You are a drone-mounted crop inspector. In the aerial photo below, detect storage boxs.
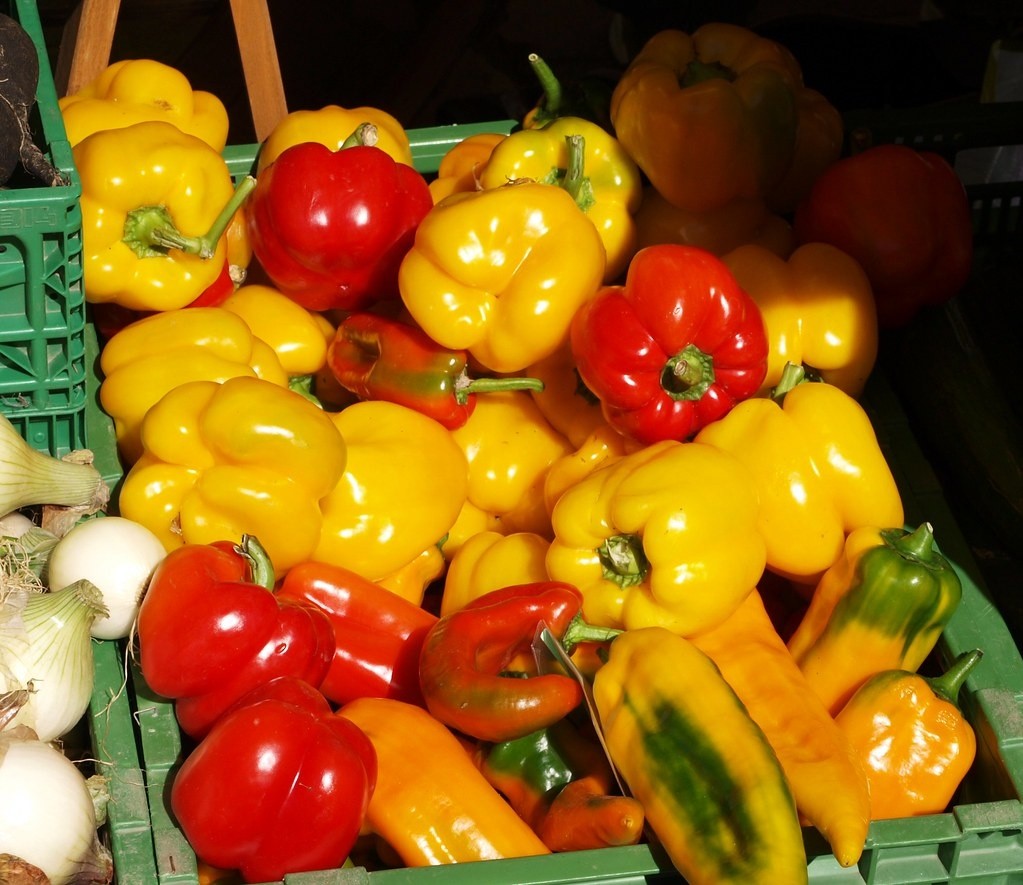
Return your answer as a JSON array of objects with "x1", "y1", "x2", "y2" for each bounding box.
[{"x1": 1, "y1": 1, "x2": 1023, "y2": 885}]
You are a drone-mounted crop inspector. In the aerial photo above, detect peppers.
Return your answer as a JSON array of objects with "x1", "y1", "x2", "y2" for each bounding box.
[{"x1": 50, "y1": 19, "x2": 982, "y2": 885}]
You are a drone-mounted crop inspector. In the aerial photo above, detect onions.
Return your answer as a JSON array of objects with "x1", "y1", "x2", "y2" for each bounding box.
[{"x1": 0, "y1": 413, "x2": 173, "y2": 885}]
